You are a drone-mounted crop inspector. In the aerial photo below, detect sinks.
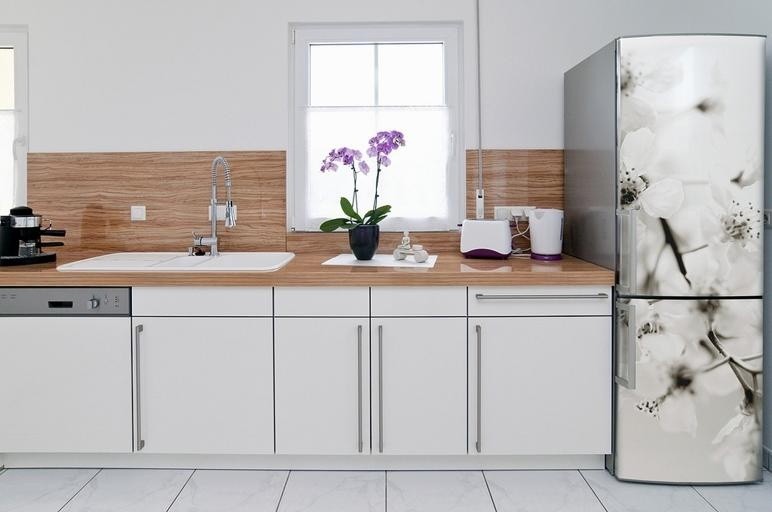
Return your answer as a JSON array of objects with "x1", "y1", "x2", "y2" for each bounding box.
[{"x1": 55, "y1": 251, "x2": 296, "y2": 274}]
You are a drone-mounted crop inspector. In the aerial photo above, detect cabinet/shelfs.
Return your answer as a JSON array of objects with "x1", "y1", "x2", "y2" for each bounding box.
[
  {"x1": 0, "y1": 270, "x2": 127, "y2": 470},
  {"x1": 273, "y1": 272, "x2": 466, "y2": 459},
  {"x1": 128, "y1": 271, "x2": 278, "y2": 463},
  {"x1": 468, "y1": 273, "x2": 614, "y2": 459}
]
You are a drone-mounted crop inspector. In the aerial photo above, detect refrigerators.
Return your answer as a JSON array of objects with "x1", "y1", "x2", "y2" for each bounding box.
[{"x1": 563, "y1": 34, "x2": 766, "y2": 487}]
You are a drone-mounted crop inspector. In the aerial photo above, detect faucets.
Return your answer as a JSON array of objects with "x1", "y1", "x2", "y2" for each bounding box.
[{"x1": 189, "y1": 155, "x2": 235, "y2": 257}]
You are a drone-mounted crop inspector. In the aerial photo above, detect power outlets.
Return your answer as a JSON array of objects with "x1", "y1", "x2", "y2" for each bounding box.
[
  {"x1": 509, "y1": 207, "x2": 523, "y2": 222},
  {"x1": 130, "y1": 206, "x2": 147, "y2": 221},
  {"x1": 494, "y1": 207, "x2": 510, "y2": 221},
  {"x1": 209, "y1": 205, "x2": 238, "y2": 221},
  {"x1": 522, "y1": 206, "x2": 536, "y2": 221}
]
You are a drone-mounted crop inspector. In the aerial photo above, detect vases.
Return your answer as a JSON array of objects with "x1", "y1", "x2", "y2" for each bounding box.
[{"x1": 348, "y1": 225, "x2": 380, "y2": 261}]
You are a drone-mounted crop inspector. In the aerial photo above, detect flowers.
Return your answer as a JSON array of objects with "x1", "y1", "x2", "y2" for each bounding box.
[{"x1": 318, "y1": 131, "x2": 406, "y2": 232}]
